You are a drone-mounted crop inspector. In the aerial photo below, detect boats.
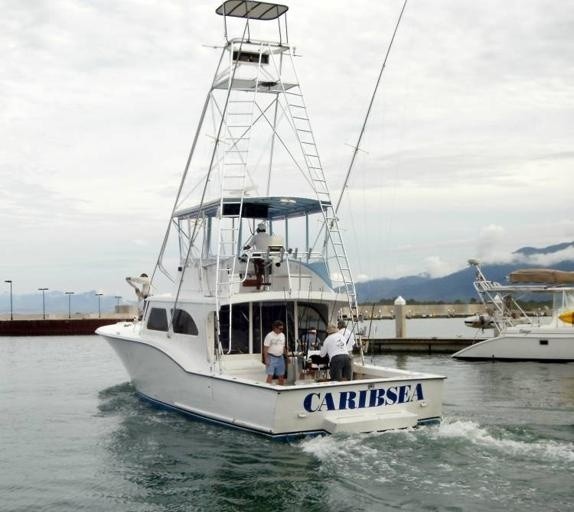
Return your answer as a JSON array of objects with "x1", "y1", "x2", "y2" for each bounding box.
[
  {"x1": 93, "y1": 0, "x2": 449, "y2": 444},
  {"x1": 450, "y1": 258, "x2": 574, "y2": 363}
]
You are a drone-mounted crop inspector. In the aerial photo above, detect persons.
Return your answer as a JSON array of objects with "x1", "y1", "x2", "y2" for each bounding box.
[
  {"x1": 337, "y1": 319, "x2": 357, "y2": 380},
  {"x1": 320, "y1": 323, "x2": 353, "y2": 381},
  {"x1": 244, "y1": 222, "x2": 272, "y2": 288},
  {"x1": 301, "y1": 327, "x2": 321, "y2": 353},
  {"x1": 125, "y1": 273, "x2": 151, "y2": 312},
  {"x1": 264, "y1": 320, "x2": 288, "y2": 385}
]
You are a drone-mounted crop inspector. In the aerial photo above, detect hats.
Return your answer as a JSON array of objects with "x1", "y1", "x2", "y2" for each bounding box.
[
  {"x1": 257, "y1": 223, "x2": 267, "y2": 230},
  {"x1": 337, "y1": 320, "x2": 347, "y2": 328},
  {"x1": 326, "y1": 324, "x2": 339, "y2": 334}
]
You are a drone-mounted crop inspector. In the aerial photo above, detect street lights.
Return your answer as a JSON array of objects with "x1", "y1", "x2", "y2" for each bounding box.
[{"x1": 4, "y1": 280, "x2": 123, "y2": 320}]
